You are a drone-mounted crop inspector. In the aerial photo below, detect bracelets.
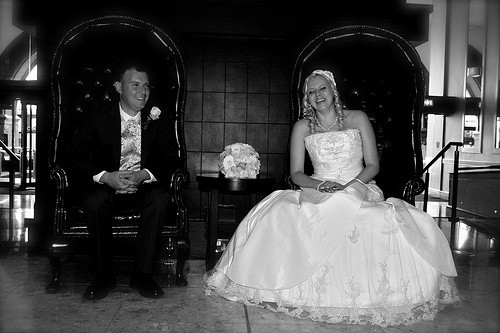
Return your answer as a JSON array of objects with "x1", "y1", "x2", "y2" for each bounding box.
[{"x1": 316, "y1": 181, "x2": 326, "y2": 191}]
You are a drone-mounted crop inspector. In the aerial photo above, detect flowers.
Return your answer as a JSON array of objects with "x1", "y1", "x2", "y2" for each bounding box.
[{"x1": 218, "y1": 142, "x2": 261, "y2": 179}]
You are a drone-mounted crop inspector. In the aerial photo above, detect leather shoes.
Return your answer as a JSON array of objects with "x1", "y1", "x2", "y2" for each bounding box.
[
  {"x1": 128, "y1": 278, "x2": 164, "y2": 299},
  {"x1": 83, "y1": 277, "x2": 116, "y2": 301}
]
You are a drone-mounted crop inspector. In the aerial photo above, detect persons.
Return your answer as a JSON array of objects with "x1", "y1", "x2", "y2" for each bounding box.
[
  {"x1": 206, "y1": 70, "x2": 460, "y2": 327},
  {"x1": 79, "y1": 66, "x2": 173, "y2": 303}
]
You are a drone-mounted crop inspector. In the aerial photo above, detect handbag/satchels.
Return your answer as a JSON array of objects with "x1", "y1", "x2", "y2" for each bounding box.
[{"x1": 367, "y1": 180, "x2": 385, "y2": 202}]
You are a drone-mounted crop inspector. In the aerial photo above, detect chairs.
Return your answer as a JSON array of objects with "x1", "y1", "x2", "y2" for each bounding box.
[
  {"x1": 278, "y1": 22, "x2": 426, "y2": 206},
  {"x1": 33, "y1": 15, "x2": 191, "y2": 287}
]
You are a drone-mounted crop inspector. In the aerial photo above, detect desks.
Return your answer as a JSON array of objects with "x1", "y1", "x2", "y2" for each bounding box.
[{"x1": 196, "y1": 172, "x2": 275, "y2": 278}]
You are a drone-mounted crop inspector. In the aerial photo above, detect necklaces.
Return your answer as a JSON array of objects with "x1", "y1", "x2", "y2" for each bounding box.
[{"x1": 316, "y1": 117, "x2": 338, "y2": 131}]
